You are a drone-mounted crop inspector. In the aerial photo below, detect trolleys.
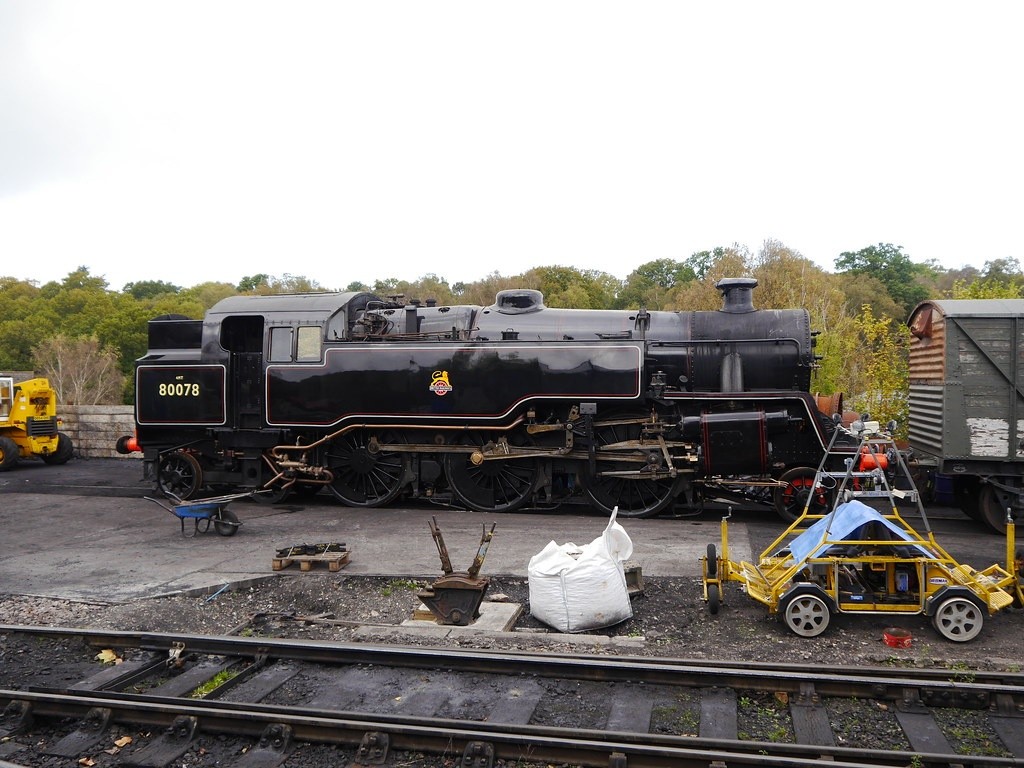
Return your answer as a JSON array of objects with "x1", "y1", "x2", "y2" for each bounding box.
[{"x1": 143, "y1": 487, "x2": 273, "y2": 535}]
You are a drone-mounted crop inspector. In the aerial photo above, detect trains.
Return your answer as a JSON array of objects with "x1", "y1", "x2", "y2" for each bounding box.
[{"x1": 115, "y1": 277, "x2": 1024, "y2": 526}]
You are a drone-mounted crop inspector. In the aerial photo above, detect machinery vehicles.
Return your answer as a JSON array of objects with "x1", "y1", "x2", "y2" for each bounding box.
[{"x1": 0, "y1": 370, "x2": 76, "y2": 473}]
